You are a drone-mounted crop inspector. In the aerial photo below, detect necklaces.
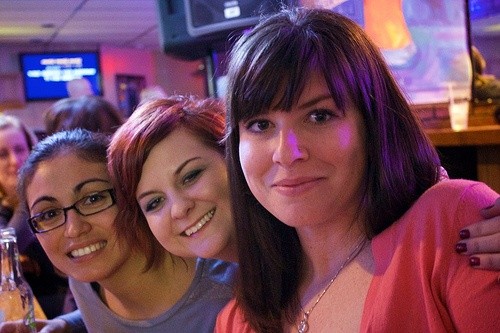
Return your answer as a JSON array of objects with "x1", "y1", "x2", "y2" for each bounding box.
[{"x1": 293, "y1": 237, "x2": 366, "y2": 333}]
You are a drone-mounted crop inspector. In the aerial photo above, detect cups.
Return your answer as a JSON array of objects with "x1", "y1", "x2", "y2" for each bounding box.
[{"x1": 449, "y1": 82, "x2": 470, "y2": 131}]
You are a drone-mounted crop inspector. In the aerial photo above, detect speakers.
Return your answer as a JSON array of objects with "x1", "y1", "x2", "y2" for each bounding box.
[{"x1": 157, "y1": 0, "x2": 300, "y2": 62}]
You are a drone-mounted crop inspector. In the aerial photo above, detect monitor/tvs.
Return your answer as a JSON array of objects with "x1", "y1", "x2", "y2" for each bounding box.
[{"x1": 19, "y1": 50, "x2": 103, "y2": 102}]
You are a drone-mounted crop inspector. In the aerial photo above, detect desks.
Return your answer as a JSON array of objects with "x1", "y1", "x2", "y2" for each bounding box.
[
  {"x1": 425, "y1": 124, "x2": 500, "y2": 194},
  {"x1": 0, "y1": 255, "x2": 48, "y2": 321}
]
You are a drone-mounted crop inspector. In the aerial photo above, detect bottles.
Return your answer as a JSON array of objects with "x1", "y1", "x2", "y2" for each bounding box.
[{"x1": 0, "y1": 228, "x2": 36, "y2": 333}]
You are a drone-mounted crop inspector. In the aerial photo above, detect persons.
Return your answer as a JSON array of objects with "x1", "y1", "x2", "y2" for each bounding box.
[
  {"x1": 0, "y1": 95, "x2": 500, "y2": 333},
  {"x1": 213, "y1": 5, "x2": 500, "y2": 333}
]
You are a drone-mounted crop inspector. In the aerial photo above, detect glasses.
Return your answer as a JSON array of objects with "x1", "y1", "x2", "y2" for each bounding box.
[{"x1": 25, "y1": 186, "x2": 121, "y2": 234}]
